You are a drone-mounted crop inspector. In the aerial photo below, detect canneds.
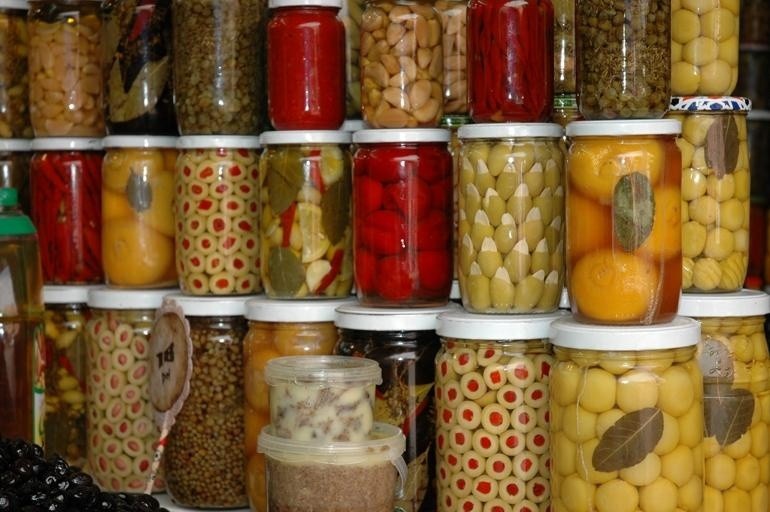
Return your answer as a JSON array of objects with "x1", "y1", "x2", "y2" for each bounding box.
[{"x1": 0, "y1": 0, "x2": 770, "y2": 511}]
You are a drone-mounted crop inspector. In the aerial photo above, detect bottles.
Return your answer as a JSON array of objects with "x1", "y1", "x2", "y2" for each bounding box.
[{"x1": 1, "y1": 184, "x2": 49, "y2": 457}]
